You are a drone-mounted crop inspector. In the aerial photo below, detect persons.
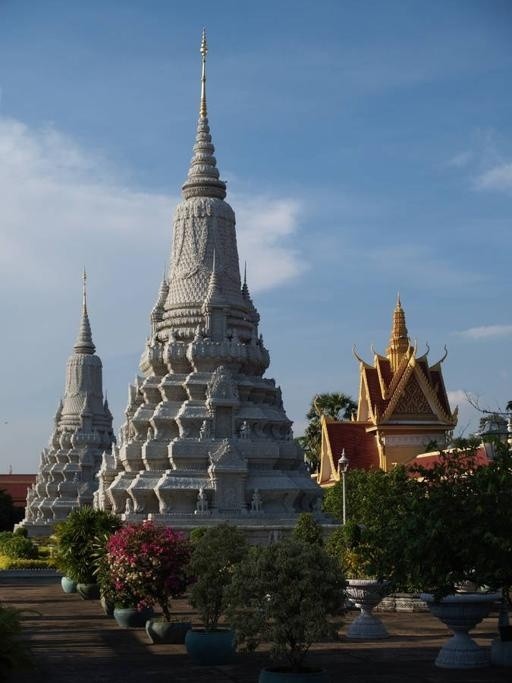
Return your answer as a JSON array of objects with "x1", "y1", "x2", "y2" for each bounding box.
[
  {"x1": 240, "y1": 421, "x2": 249, "y2": 438},
  {"x1": 251, "y1": 489, "x2": 263, "y2": 511},
  {"x1": 200, "y1": 420, "x2": 210, "y2": 438},
  {"x1": 197, "y1": 488, "x2": 209, "y2": 512}
]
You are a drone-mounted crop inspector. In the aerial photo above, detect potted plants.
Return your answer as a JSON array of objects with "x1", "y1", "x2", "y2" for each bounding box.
[
  {"x1": 47, "y1": 511, "x2": 345, "y2": 681},
  {"x1": 325, "y1": 438, "x2": 511, "y2": 669}
]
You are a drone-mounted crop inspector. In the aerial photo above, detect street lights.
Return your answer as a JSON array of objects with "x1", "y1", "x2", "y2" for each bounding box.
[
  {"x1": 478, "y1": 411, "x2": 512, "y2": 471},
  {"x1": 335, "y1": 447, "x2": 352, "y2": 525}
]
